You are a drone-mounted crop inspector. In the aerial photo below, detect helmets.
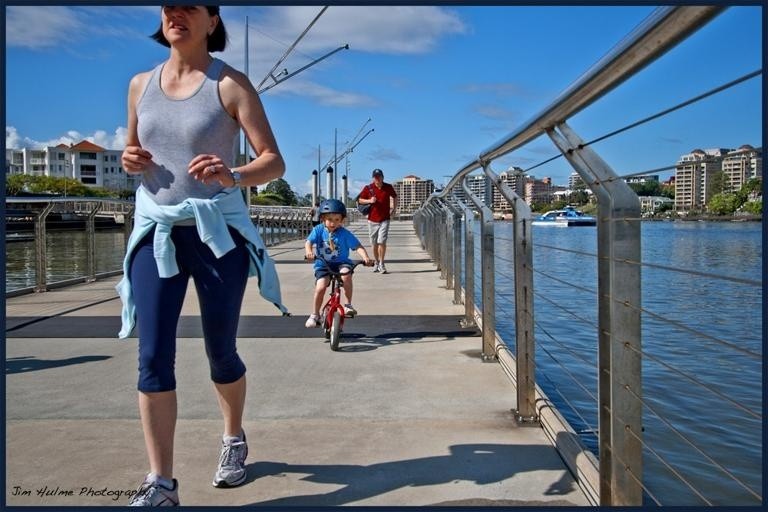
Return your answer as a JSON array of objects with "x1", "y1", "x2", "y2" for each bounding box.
[{"x1": 319, "y1": 199, "x2": 347, "y2": 220}]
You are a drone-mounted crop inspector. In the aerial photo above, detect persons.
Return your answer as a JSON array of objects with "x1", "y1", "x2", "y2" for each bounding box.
[
  {"x1": 310, "y1": 202, "x2": 321, "y2": 228},
  {"x1": 121, "y1": 2, "x2": 286, "y2": 507},
  {"x1": 355, "y1": 169, "x2": 397, "y2": 274},
  {"x1": 304, "y1": 199, "x2": 371, "y2": 328}
]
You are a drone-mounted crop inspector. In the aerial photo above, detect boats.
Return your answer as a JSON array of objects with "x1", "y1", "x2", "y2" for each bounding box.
[{"x1": 528, "y1": 206, "x2": 597, "y2": 228}]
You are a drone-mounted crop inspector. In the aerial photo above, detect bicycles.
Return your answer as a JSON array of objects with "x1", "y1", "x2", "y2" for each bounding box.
[{"x1": 304, "y1": 255, "x2": 375, "y2": 352}]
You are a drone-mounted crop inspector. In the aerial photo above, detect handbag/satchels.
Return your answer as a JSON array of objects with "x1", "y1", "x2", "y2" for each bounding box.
[{"x1": 355, "y1": 197, "x2": 373, "y2": 214}]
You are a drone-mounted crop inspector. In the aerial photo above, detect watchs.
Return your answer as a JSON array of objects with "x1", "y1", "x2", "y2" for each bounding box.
[{"x1": 228, "y1": 167, "x2": 241, "y2": 188}]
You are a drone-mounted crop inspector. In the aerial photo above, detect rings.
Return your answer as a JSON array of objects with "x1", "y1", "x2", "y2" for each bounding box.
[{"x1": 210, "y1": 165, "x2": 216, "y2": 174}]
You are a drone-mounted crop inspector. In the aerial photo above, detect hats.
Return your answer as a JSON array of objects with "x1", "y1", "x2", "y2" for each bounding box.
[{"x1": 372, "y1": 170, "x2": 383, "y2": 177}]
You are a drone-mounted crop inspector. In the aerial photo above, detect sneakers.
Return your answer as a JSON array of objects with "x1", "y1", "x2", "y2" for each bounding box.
[
  {"x1": 214, "y1": 429, "x2": 248, "y2": 487},
  {"x1": 372, "y1": 262, "x2": 387, "y2": 274},
  {"x1": 305, "y1": 314, "x2": 320, "y2": 328},
  {"x1": 344, "y1": 304, "x2": 357, "y2": 315},
  {"x1": 130, "y1": 479, "x2": 180, "y2": 507}
]
